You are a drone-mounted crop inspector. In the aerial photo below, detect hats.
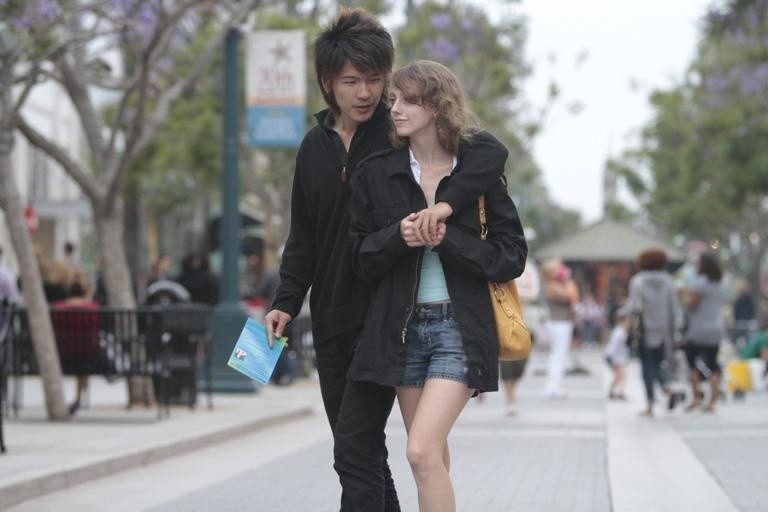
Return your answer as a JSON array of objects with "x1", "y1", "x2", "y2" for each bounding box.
[{"x1": 539, "y1": 259, "x2": 571, "y2": 283}]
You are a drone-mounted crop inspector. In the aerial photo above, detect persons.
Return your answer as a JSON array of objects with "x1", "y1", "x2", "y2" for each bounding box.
[
  {"x1": 262, "y1": 5, "x2": 511, "y2": 511},
  {"x1": 151, "y1": 249, "x2": 279, "y2": 304},
  {"x1": 338, "y1": 59, "x2": 532, "y2": 510},
  {"x1": 0, "y1": 243, "x2": 115, "y2": 413},
  {"x1": 500, "y1": 250, "x2": 768, "y2": 412}
]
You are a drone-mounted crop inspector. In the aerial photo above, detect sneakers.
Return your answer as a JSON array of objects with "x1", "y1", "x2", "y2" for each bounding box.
[{"x1": 605, "y1": 388, "x2": 719, "y2": 418}]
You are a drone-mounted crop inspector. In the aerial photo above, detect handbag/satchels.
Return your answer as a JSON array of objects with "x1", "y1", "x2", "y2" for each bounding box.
[
  {"x1": 475, "y1": 191, "x2": 534, "y2": 364},
  {"x1": 628, "y1": 313, "x2": 646, "y2": 357}
]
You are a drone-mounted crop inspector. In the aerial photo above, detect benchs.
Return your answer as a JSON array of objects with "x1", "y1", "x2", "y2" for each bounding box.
[
  {"x1": 270, "y1": 313, "x2": 313, "y2": 384},
  {"x1": 2, "y1": 306, "x2": 219, "y2": 418}
]
